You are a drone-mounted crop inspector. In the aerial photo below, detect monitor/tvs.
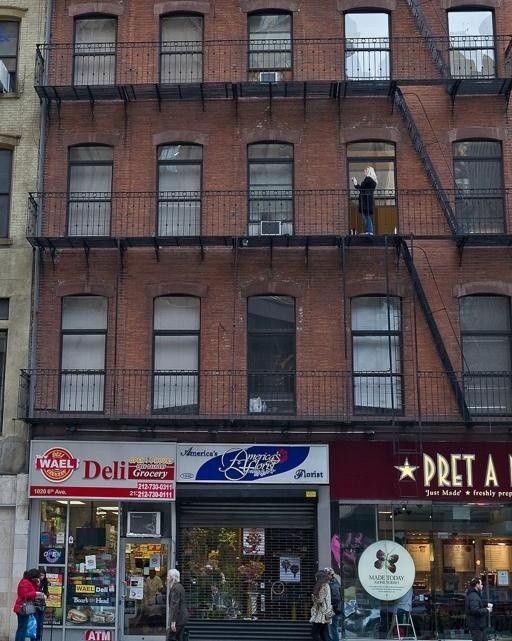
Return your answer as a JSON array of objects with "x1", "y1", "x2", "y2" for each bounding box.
[
  {"x1": 125, "y1": 512, "x2": 162, "y2": 537},
  {"x1": 76, "y1": 526, "x2": 106, "y2": 547}
]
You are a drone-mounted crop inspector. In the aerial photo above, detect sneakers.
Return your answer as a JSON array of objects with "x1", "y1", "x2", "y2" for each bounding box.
[{"x1": 359, "y1": 231, "x2": 374, "y2": 236}]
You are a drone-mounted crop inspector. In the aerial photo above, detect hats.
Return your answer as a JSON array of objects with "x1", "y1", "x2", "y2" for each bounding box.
[{"x1": 324, "y1": 567, "x2": 334, "y2": 575}]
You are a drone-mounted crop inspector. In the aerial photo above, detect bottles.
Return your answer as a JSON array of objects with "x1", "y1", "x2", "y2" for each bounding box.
[{"x1": 393, "y1": 226, "x2": 398, "y2": 234}]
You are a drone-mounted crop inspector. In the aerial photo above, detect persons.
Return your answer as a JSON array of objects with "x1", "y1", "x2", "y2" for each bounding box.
[
  {"x1": 309, "y1": 571, "x2": 336, "y2": 641},
  {"x1": 146, "y1": 570, "x2": 165, "y2": 633},
  {"x1": 351, "y1": 166, "x2": 378, "y2": 235},
  {"x1": 30, "y1": 567, "x2": 50, "y2": 641},
  {"x1": 397, "y1": 586, "x2": 413, "y2": 624},
  {"x1": 166, "y1": 569, "x2": 189, "y2": 641},
  {"x1": 13, "y1": 568, "x2": 47, "y2": 641},
  {"x1": 465, "y1": 577, "x2": 493, "y2": 641},
  {"x1": 324, "y1": 567, "x2": 345, "y2": 641}
]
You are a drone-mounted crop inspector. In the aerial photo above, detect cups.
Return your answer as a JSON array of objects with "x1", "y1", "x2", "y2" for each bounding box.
[{"x1": 488, "y1": 602, "x2": 494, "y2": 608}]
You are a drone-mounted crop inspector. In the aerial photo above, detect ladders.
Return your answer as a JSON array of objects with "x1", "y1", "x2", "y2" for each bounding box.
[{"x1": 395, "y1": 613, "x2": 417, "y2": 641}]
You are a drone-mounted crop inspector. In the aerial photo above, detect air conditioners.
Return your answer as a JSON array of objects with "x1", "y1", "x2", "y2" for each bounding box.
[
  {"x1": 260, "y1": 72, "x2": 278, "y2": 85},
  {"x1": 260, "y1": 221, "x2": 281, "y2": 235}
]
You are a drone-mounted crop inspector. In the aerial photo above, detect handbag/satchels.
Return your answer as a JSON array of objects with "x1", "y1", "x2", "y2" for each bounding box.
[
  {"x1": 25, "y1": 614, "x2": 37, "y2": 639},
  {"x1": 21, "y1": 602, "x2": 36, "y2": 615}
]
